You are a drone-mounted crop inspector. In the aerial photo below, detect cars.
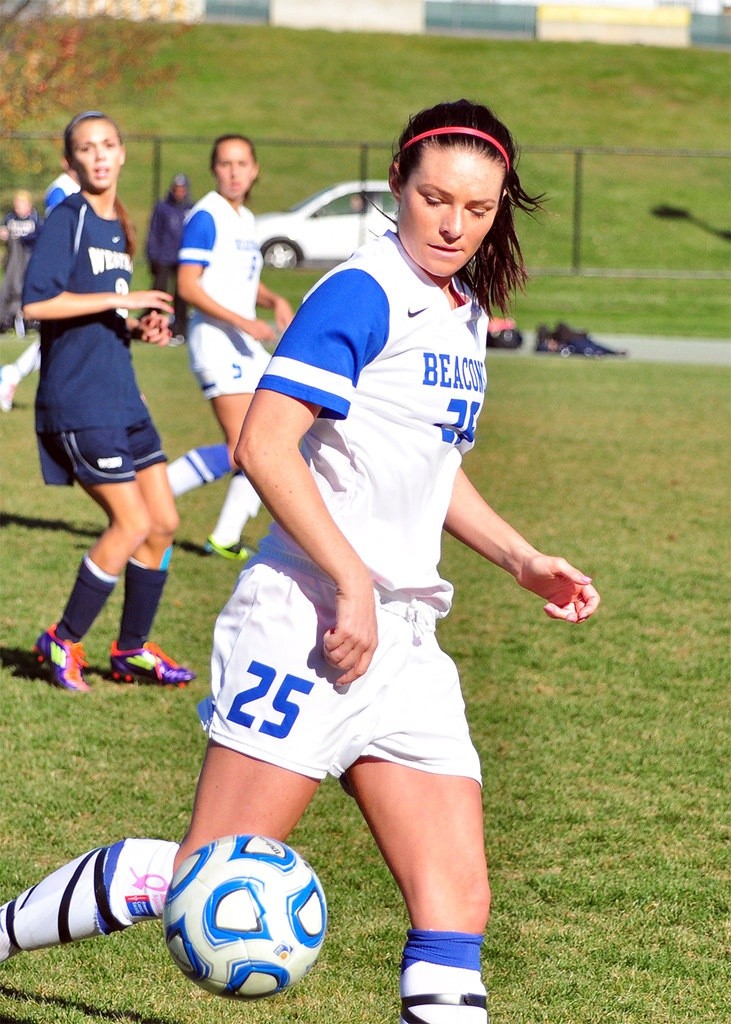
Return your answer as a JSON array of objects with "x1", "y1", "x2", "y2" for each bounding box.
[{"x1": 253, "y1": 179, "x2": 399, "y2": 268}]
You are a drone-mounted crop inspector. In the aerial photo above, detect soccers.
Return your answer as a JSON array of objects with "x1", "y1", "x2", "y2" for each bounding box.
[{"x1": 164, "y1": 834, "x2": 325, "y2": 999}]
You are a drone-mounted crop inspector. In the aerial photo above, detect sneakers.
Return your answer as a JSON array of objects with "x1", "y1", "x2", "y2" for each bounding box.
[
  {"x1": 205, "y1": 536, "x2": 255, "y2": 562},
  {"x1": 110, "y1": 640, "x2": 196, "y2": 686},
  {"x1": 34, "y1": 624, "x2": 88, "y2": 690},
  {"x1": 1, "y1": 364, "x2": 21, "y2": 413}
]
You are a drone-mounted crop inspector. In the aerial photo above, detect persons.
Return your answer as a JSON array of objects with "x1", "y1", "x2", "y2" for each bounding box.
[
  {"x1": 0, "y1": 148, "x2": 82, "y2": 413},
  {"x1": 0, "y1": 188, "x2": 41, "y2": 331},
  {"x1": 146, "y1": 172, "x2": 196, "y2": 341},
  {"x1": 349, "y1": 193, "x2": 363, "y2": 213},
  {"x1": 164, "y1": 136, "x2": 295, "y2": 563},
  {"x1": 0, "y1": 98, "x2": 602, "y2": 1024},
  {"x1": 21, "y1": 110, "x2": 196, "y2": 692}
]
